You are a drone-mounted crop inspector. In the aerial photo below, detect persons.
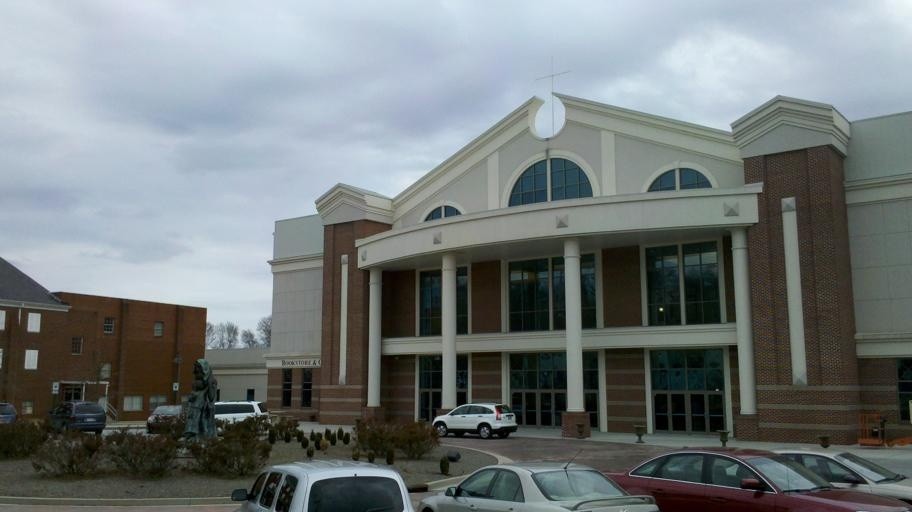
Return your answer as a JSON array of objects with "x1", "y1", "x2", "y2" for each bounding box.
[{"x1": 181, "y1": 359, "x2": 218, "y2": 442}]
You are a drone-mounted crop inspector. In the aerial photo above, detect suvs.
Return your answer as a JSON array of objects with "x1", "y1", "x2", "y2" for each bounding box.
[
  {"x1": 426, "y1": 401, "x2": 519, "y2": 439},
  {"x1": 46, "y1": 400, "x2": 106, "y2": 437}
]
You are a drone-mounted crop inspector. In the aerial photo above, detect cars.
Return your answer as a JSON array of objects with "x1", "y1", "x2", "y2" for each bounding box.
[
  {"x1": 776, "y1": 446, "x2": 912, "y2": 505},
  {"x1": 602, "y1": 445, "x2": 912, "y2": 510},
  {"x1": 0, "y1": 402, "x2": 20, "y2": 424},
  {"x1": 416, "y1": 449, "x2": 658, "y2": 511},
  {"x1": 148, "y1": 405, "x2": 184, "y2": 434}
]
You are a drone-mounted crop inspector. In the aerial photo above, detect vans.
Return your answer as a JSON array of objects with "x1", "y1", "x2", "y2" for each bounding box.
[
  {"x1": 214, "y1": 399, "x2": 271, "y2": 436},
  {"x1": 228, "y1": 458, "x2": 430, "y2": 512}
]
